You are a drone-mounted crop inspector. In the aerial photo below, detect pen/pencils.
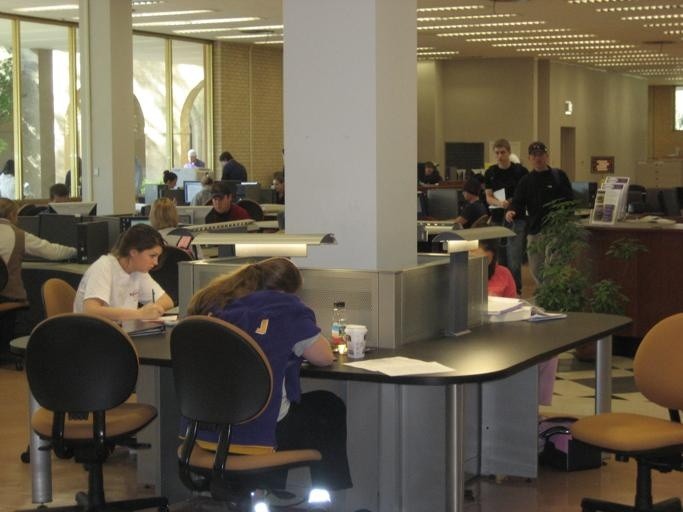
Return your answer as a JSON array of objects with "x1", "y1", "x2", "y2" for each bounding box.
[{"x1": 152, "y1": 289, "x2": 155, "y2": 303}]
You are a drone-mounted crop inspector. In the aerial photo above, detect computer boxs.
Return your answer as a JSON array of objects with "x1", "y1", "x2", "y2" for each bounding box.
[
  {"x1": 427, "y1": 189, "x2": 463, "y2": 218},
  {"x1": 77, "y1": 221, "x2": 107, "y2": 264},
  {"x1": 145, "y1": 184, "x2": 169, "y2": 204}
]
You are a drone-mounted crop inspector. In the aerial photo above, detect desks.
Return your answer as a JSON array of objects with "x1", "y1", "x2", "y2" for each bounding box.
[{"x1": 10, "y1": 312, "x2": 639, "y2": 512}]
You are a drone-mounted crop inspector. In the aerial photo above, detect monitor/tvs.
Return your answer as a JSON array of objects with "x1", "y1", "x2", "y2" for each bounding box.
[
  {"x1": 38, "y1": 214, "x2": 79, "y2": 246},
  {"x1": 184, "y1": 180, "x2": 203, "y2": 203},
  {"x1": 241, "y1": 181, "x2": 261, "y2": 189},
  {"x1": 49, "y1": 202, "x2": 98, "y2": 218},
  {"x1": 160, "y1": 189, "x2": 187, "y2": 206},
  {"x1": 445, "y1": 141, "x2": 484, "y2": 170},
  {"x1": 417, "y1": 192, "x2": 424, "y2": 215},
  {"x1": 120, "y1": 217, "x2": 151, "y2": 234}
]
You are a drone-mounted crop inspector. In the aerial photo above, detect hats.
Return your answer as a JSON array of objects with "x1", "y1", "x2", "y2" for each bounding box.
[
  {"x1": 208, "y1": 184, "x2": 228, "y2": 199},
  {"x1": 528, "y1": 142, "x2": 548, "y2": 153}
]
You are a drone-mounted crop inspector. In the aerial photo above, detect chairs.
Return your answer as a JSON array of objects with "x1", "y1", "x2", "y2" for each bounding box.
[
  {"x1": 20, "y1": 278, "x2": 137, "y2": 464},
  {"x1": 570, "y1": 311, "x2": 683, "y2": 511},
  {"x1": 23, "y1": 312, "x2": 169, "y2": 512},
  {"x1": 0, "y1": 182, "x2": 285, "y2": 371},
  {"x1": 417, "y1": 169, "x2": 683, "y2": 235},
  {"x1": 170, "y1": 316, "x2": 322, "y2": 511}
]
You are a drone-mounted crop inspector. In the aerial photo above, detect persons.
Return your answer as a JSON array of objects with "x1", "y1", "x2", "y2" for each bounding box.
[
  {"x1": 177, "y1": 256, "x2": 354, "y2": 512},
  {"x1": 417, "y1": 161, "x2": 442, "y2": 187},
  {"x1": 190, "y1": 176, "x2": 214, "y2": 206},
  {"x1": 49, "y1": 183, "x2": 70, "y2": 203},
  {"x1": 0, "y1": 159, "x2": 16, "y2": 199},
  {"x1": 147, "y1": 197, "x2": 179, "y2": 245},
  {"x1": 271, "y1": 170, "x2": 284, "y2": 204},
  {"x1": 72, "y1": 223, "x2": 173, "y2": 327},
  {"x1": 219, "y1": 151, "x2": 247, "y2": 183},
  {"x1": 162, "y1": 170, "x2": 177, "y2": 189},
  {"x1": 0, "y1": 199, "x2": 82, "y2": 347},
  {"x1": 64, "y1": 156, "x2": 81, "y2": 195},
  {"x1": 454, "y1": 138, "x2": 572, "y2": 298},
  {"x1": 204, "y1": 180, "x2": 250, "y2": 224},
  {"x1": 183, "y1": 149, "x2": 205, "y2": 168}
]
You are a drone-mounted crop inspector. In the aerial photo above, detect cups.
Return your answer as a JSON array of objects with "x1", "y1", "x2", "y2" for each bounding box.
[
  {"x1": 345, "y1": 322, "x2": 369, "y2": 359},
  {"x1": 457, "y1": 168, "x2": 468, "y2": 181}
]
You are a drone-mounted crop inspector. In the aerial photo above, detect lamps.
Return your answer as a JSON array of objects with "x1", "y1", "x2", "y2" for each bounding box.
[
  {"x1": 191, "y1": 232, "x2": 336, "y2": 259},
  {"x1": 430, "y1": 226, "x2": 517, "y2": 336}
]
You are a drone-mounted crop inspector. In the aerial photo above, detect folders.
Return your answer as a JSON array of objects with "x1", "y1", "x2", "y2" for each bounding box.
[{"x1": 120, "y1": 320, "x2": 166, "y2": 337}]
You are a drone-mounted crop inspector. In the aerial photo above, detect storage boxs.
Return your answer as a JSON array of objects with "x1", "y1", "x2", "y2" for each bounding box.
[{"x1": 538, "y1": 417, "x2": 601, "y2": 472}]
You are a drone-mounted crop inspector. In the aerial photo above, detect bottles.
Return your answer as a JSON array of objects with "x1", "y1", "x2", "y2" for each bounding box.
[{"x1": 331, "y1": 299, "x2": 346, "y2": 351}]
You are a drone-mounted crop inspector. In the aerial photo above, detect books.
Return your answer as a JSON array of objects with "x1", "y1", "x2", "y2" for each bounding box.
[
  {"x1": 600, "y1": 181, "x2": 625, "y2": 220},
  {"x1": 606, "y1": 176, "x2": 630, "y2": 218},
  {"x1": 591, "y1": 189, "x2": 620, "y2": 225},
  {"x1": 485, "y1": 295, "x2": 531, "y2": 315},
  {"x1": 488, "y1": 305, "x2": 547, "y2": 321},
  {"x1": 121, "y1": 317, "x2": 166, "y2": 336},
  {"x1": 529, "y1": 312, "x2": 567, "y2": 321}
]
